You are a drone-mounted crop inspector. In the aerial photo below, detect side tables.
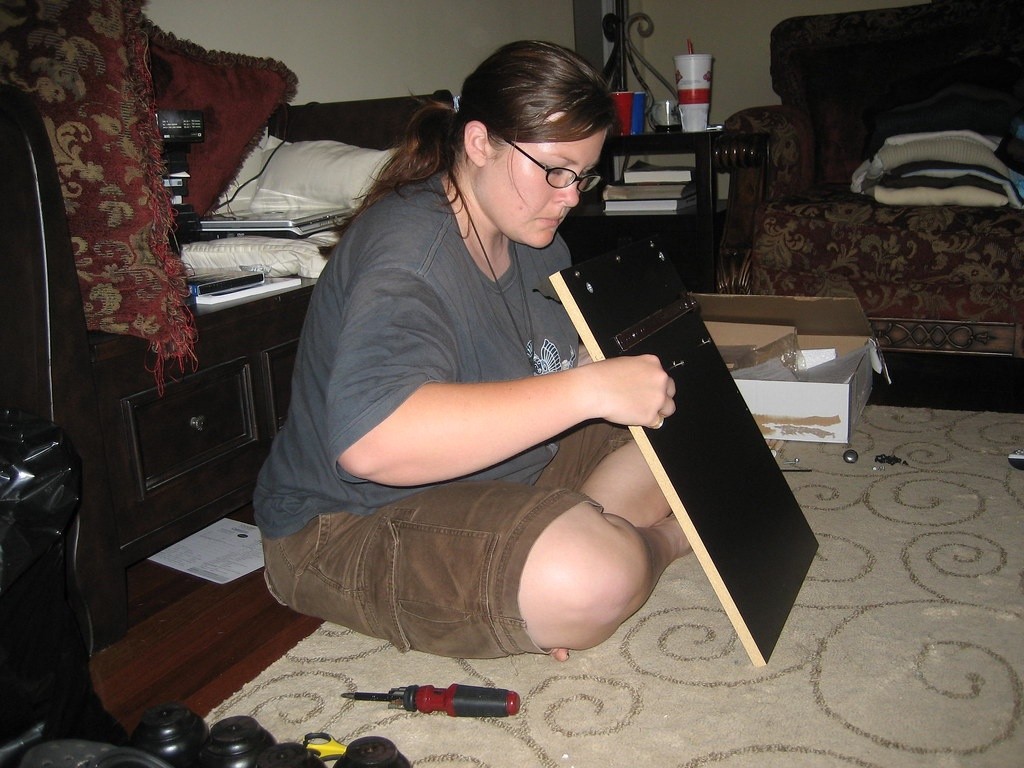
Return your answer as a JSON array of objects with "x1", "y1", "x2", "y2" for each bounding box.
[{"x1": 558, "y1": 131, "x2": 725, "y2": 293}]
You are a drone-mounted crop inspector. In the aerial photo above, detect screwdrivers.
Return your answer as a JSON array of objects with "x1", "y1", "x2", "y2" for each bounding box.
[{"x1": 340, "y1": 683, "x2": 522, "y2": 719}]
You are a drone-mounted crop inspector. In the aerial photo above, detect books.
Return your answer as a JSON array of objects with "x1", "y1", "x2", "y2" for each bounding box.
[{"x1": 603, "y1": 161, "x2": 697, "y2": 211}]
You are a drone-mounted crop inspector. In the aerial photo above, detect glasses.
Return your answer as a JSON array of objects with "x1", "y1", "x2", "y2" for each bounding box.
[{"x1": 489, "y1": 125, "x2": 602, "y2": 192}]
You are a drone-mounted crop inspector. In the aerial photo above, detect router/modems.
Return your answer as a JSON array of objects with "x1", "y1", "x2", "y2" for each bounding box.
[{"x1": 187, "y1": 269, "x2": 264, "y2": 297}]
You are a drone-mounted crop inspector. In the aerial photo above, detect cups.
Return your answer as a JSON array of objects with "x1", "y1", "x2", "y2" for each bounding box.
[
  {"x1": 649, "y1": 101, "x2": 681, "y2": 131},
  {"x1": 673, "y1": 53, "x2": 712, "y2": 133},
  {"x1": 608, "y1": 91, "x2": 634, "y2": 136},
  {"x1": 629, "y1": 91, "x2": 654, "y2": 135}
]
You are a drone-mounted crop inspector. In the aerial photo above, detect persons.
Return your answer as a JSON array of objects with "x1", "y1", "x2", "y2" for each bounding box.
[{"x1": 252, "y1": 39, "x2": 691, "y2": 658}]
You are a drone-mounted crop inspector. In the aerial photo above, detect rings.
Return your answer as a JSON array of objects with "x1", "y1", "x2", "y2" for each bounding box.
[{"x1": 651, "y1": 417, "x2": 663, "y2": 428}]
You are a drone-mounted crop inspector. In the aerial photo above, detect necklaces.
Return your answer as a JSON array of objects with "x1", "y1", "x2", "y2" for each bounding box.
[{"x1": 456, "y1": 184, "x2": 536, "y2": 373}]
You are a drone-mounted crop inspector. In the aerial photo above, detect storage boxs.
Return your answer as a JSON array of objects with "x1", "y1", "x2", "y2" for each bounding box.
[{"x1": 704, "y1": 321, "x2": 874, "y2": 445}]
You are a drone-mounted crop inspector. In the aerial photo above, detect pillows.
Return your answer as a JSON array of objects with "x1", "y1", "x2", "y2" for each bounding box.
[
  {"x1": 144, "y1": 21, "x2": 300, "y2": 220},
  {"x1": 250, "y1": 138, "x2": 397, "y2": 209},
  {"x1": 0, "y1": 0, "x2": 200, "y2": 397}
]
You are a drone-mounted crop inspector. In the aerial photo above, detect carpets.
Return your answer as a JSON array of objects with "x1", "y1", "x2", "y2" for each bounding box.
[{"x1": 204, "y1": 404, "x2": 1024, "y2": 768}]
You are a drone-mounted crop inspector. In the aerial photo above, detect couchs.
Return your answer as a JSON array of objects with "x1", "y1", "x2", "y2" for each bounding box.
[
  {"x1": 0, "y1": 85, "x2": 454, "y2": 661},
  {"x1": 712, "y1": 0, "x2": 1024, "y2": 413}
]
